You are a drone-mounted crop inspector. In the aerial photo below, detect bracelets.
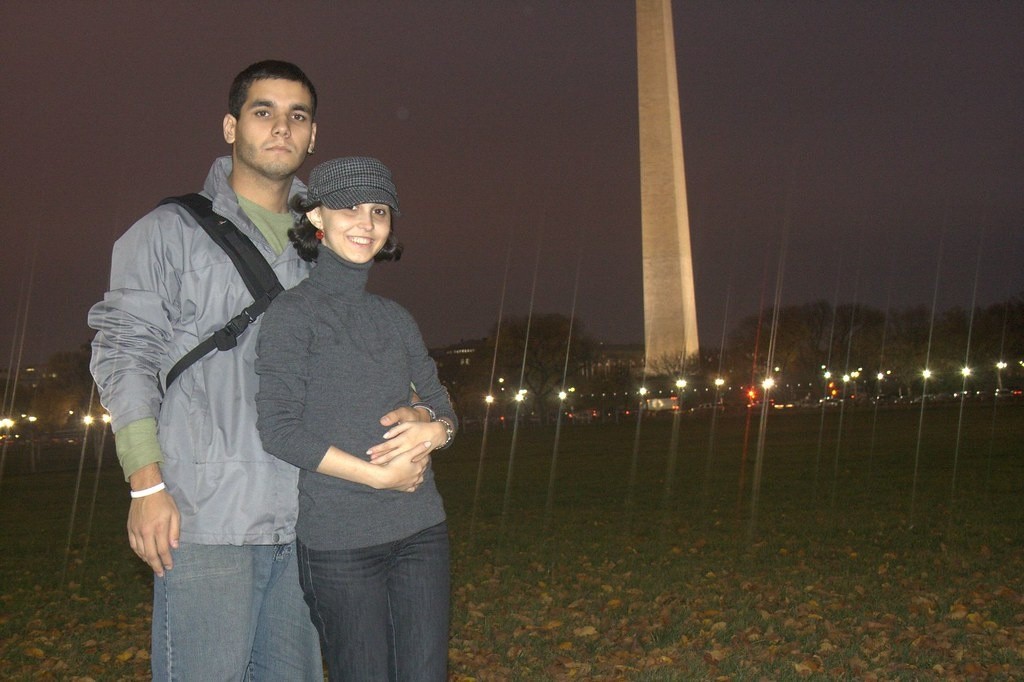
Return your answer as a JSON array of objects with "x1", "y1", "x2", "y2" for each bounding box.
[
  {"x1": 412, "y1": 403, "x2": 436, "y2": 419},
  {"x1": 435, "y1": 419, "x2": 453, "y2": 450},
  {"x1": 131, "y1": 483, "x2": 166, "y2": 498}
]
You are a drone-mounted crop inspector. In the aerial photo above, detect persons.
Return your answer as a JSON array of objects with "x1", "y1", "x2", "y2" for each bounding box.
[
  {"x1": 86, "y1": 61, "x2": 432, "y2": 681},
  {"x1": 255, "y1": 157, "x2": 460, "y2": 682}
]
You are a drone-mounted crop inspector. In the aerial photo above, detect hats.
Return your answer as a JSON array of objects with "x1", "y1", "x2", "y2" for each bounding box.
[{"x1": 305, "y1": 156, "x2": 401, "y2": 217}]
